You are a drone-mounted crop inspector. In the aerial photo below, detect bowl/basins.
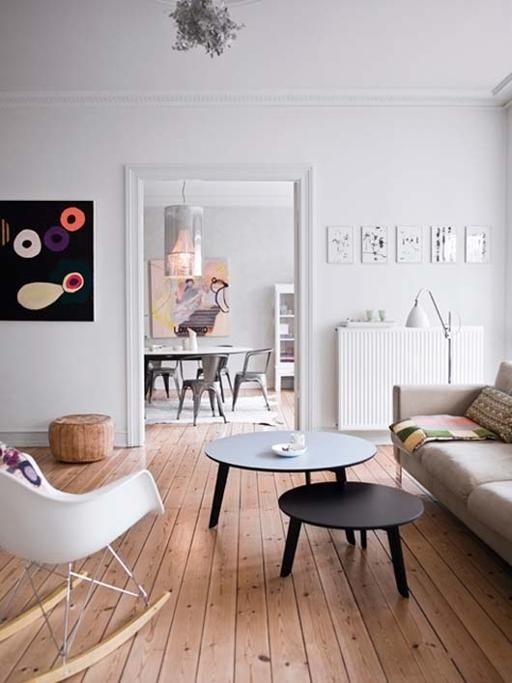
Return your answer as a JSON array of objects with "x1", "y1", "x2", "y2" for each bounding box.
[{"x1": 173, "y1": 345, "x2": 182, "y2": 350}]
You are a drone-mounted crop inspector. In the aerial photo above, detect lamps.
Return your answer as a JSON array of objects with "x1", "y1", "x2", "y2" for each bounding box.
[
  {"x1": 164, "y1": 180, "x2": 203, "y2": 279},
  {"x1": 405, "y1": 287, "x2": 452, "y2": 384}
]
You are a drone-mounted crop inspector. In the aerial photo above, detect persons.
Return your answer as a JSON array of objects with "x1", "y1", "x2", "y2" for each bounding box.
[{"x1": 172, "y1": 279, "x2": 202, "y2": 326}]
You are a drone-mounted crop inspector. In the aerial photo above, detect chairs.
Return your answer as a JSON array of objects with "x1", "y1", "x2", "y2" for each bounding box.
[
  {"x1": 144, "y1": 345, "x2": 273, "y2": 426},
  {"x1": 0, "y1": 452, "x2": 171, "y2": 683}
]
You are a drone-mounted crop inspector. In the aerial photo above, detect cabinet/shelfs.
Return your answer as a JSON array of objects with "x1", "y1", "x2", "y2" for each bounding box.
[{"x1": 274, "y1": 283, "x2": 295, "y2": 393}]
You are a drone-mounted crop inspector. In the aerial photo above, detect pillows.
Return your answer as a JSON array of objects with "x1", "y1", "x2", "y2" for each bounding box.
[{"x1": 463, "y1": 387, "x2": 512, "y2": 443}]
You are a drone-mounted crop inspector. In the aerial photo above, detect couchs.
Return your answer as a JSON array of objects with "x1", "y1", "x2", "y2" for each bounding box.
[{"x1": 392, "y1": 361, "x2": 512, "y2": 568}]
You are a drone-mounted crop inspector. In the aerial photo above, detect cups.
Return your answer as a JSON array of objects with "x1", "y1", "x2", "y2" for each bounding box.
[
  {"x1": 289, "y1": 327, "x2": 293, "y2": 337},
  {"x1": 287, "y1": 432, "x2": 305, "y2": 449},
  {"x1": 288, "y1": 304, "x2": 293, "y2": 314},
  {"x1": 280, "y1": 304, "x2": 288, "y2": 315},
  {"x1": 183, "y1": 338, "x2": 189, "y2": 350},
  {"x1": 366, "y1": 310, "x2": 386, "y2": 322}
]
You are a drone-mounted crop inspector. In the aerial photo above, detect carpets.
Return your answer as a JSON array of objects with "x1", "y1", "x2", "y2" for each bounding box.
[{"x1": 144, "y1": 393, "x2": 287, "y2": 429}]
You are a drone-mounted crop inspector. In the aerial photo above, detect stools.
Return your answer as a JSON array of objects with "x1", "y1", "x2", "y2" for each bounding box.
[{"x1": 49, "y1": 413, "x2": 116, "y2": 461}]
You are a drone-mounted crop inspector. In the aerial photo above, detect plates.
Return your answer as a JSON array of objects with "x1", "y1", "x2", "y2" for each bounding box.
[{"x1": 272, "y1": 444, "x2": 308, "y2": 457}]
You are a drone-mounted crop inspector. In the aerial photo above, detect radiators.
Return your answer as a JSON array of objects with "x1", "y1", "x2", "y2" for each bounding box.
[{"x1": 337, "y1": 326, "x2": 450, "y2": 427}]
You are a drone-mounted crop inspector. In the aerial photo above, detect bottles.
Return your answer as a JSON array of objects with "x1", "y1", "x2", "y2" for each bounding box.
[{"x1": 189, "y1": 331, "x2": 198, "y2": 351}]
